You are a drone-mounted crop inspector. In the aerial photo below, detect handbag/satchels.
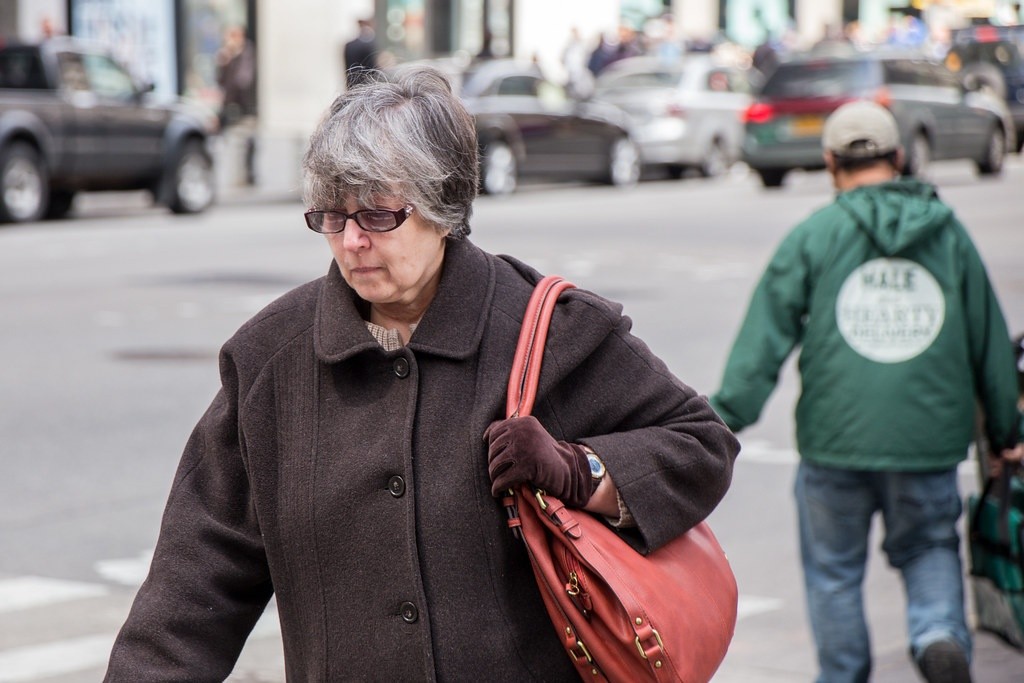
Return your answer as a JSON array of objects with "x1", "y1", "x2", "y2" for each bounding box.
[
  {"x1": 505, "y1": 274, "x2": 737, "y2": 683},
  {"x1": 967, "y1": 462, "x2": 1024, "y2": 650}
]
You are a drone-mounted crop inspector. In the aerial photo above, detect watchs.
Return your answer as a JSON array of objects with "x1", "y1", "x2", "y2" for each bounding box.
[{"x1": 584, "y1": 448, "x2": 606, "y2": 497}]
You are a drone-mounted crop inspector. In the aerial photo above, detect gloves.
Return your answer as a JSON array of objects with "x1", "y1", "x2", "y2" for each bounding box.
[{"x1": 481, "y1": 416, "x2": 593, "y2": 511}]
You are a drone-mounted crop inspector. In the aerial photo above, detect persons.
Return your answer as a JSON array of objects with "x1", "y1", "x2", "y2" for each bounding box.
[
  {"x1": 708, "y1": 100, "x2": 1024, "y2": 683},
  {"x1": 567, "y1": 16, "x2": 926, "y2": 74},
  {"x1": 345, "y1": 20, "x2": 379, "y2": 87},
  {"x1": 212, "y1": 24, "x2": 256, "y2": 188},
  {"x1": 100, "y1": 69, "x2": 742, "y2": 683}
]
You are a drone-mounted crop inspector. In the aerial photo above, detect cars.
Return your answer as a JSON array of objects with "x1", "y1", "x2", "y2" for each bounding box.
[
  {"x1": 596, "y1": 52, "x2": 765, "y2": 183},
  {"x1": 741, "y1": 52, "x2": 1019, "y2": 180},
  {"x1": 402, "y1": 63, "x2": 644, "y2": 200}
]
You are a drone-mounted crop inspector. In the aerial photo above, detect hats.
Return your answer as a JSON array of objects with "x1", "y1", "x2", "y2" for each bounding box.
[{"x1": 822, "y1": 101, "x2": 902, "y2": 159}]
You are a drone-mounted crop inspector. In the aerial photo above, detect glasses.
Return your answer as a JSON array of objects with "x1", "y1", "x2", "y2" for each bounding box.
[{"x1": 304, "y1": 201, "x2": 417, "y2": 235}]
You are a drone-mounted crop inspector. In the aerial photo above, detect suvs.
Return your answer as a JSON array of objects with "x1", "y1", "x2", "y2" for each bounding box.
[
  {"x1": 946, "y1": 26, "x2": 1024, "y2": 149},
  {"x1": 0, "y1": 38, "x2": 217, "y2": 225}
]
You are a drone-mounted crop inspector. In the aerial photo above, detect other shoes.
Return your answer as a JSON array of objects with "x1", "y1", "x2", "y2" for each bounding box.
[{"x1": 914, "y1": 639, "x2": 974, "y2": 683}]
